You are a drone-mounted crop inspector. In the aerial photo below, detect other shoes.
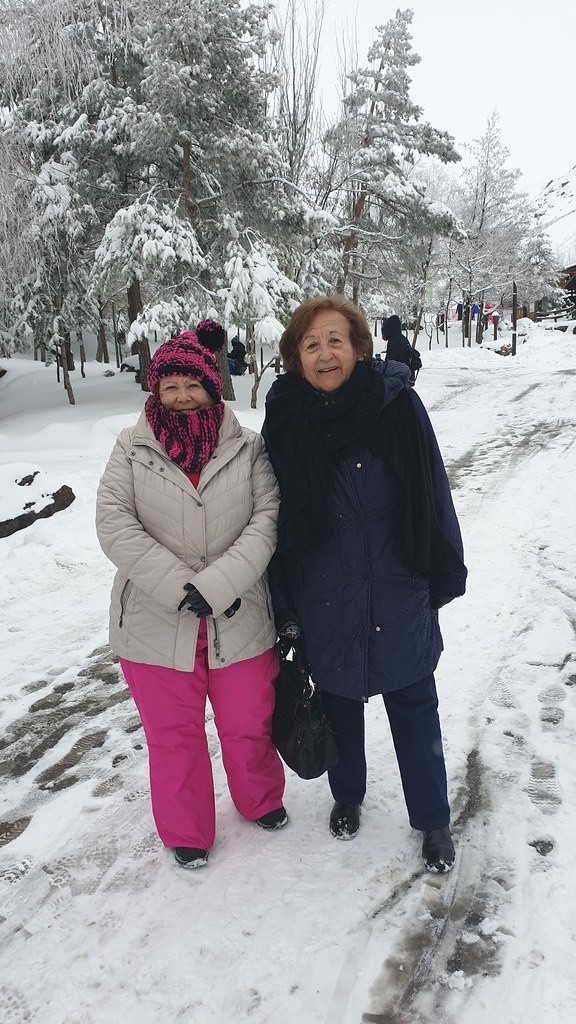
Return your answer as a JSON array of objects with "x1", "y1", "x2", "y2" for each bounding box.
[
  {"x1": 258, "y1": 806, "x2": 288, "y2": 830},
  {"x1": 174, "y1": 847, "x2": 209, "y2": 867}
]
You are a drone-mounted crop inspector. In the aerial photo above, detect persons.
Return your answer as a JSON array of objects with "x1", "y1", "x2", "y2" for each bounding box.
[
  {"x1": 438, "y1": 301, "x2": 495, "y2": 334},
  {"x1": 262, "y1": 295, "x2": 467, "y2": 875},
  {"x1": 376, "y1": 314, "x2": 419, "y2": 388},
  {"x1": 225, "y1": 335, "x2": 247, "y2": 376},
  {"x1": 95, "y1": 319, "x2": 289, "y2": 870}
]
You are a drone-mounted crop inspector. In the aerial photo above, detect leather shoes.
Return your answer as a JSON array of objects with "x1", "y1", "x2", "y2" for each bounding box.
[
  {"x1": 329, "y1": 801, "x2": 361, "y2": 839},
  {"x1": 422, "y1": 824, "x2": 455, "y2": 873}
]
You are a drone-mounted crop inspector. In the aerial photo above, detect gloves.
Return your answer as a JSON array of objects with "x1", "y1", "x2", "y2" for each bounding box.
[
  {"x1": 184, "y1": 583, "x2": 212, "y2": 618},
  {"x1": 430, "y1": 591, "x2": 458, "y2": 610},
  {"x1": 224, "y1": 598, "x2": 241, "y2": 617},
  {"x1": 280, "y1": 622, "x2": 301, "y2": 639}
]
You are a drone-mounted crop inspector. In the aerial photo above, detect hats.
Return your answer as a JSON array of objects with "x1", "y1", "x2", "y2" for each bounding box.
[
  {"x1": 147, "y1": 319, "x2": 227, "y2": 402},
  {"x1": 232, "y1": 335, "x2": 239, "y2": 342}
]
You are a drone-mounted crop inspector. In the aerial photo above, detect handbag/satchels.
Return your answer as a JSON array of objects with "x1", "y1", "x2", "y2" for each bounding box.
[
  {"x1": 401, "y1": 336, "x2": 422, "y2": 370},
  {"x1": 271, "y1": 634, "x2": 338, "y2": 780}
]
